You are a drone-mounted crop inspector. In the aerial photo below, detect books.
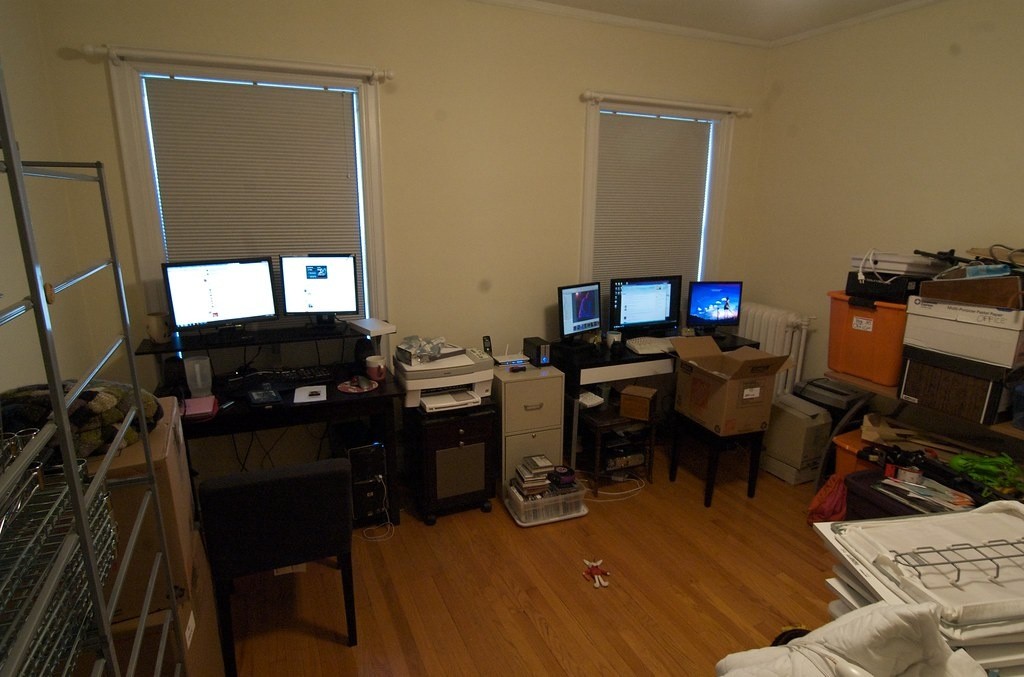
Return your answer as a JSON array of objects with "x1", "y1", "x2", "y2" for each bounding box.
[
  {"x1": 179, "y1": 395, "x2": 217, "y2": 421},
  {"x1": 512, "y1": 453, "x2": 556, "y2": 497}
]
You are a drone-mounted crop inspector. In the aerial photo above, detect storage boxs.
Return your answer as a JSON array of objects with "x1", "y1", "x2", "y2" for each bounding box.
[
  {"x1": 759, "y1": 452, "x2": 819, "y2": 487},
  {"x1": 827, "y1": 246, "x2": 1024, "y2": 422},
  {"x1": 832, "y1": 428, "x2": 881, "y2": 473},
  {"x1": 761, "y1": 391, "x2": 830, "y2": 471},
  {"x1": 845, "y1": 471, "x2": 973, "y2": 520},
  {"x1": 79, "y1": 397, "x2": 197, "y2": 677},
  {"x1": 504, "y1": 479, "x2": 585, "y2": 522},
  {"x1": 660, "y1": 337, "x2": 789, "y2": 436},
  {"x1": 620, "y1": 384, "x2": 660, "y2": 421}
]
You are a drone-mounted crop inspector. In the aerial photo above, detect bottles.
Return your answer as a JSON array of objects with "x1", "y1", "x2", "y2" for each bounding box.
[{"x1": 666, "y1": 321, "x2": 677, "y2": 337}]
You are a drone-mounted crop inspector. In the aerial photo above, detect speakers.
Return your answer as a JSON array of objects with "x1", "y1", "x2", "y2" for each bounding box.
[
  {"x1": 163, "y1": 357, "x2": 191, "y2": 405},
  {"x1": 354, "y1": 339, "x2": 376, "y2": 373},
  {"x1": 183, "y1": 355, "x2": 214, "y2": 398}
]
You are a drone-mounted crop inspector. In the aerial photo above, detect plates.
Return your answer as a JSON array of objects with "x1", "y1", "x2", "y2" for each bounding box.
[{"x1": 337, "y1": 380, "x2": 378, "y2": 393}]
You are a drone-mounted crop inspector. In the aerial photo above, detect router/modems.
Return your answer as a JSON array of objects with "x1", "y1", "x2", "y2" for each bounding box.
[{"x1": 493, "y1": 344, "x2": 530, "y2": 365}]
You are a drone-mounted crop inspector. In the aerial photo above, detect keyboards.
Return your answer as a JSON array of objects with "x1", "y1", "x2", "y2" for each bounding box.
[
  {"x1": 226, "y1": 364, "x2": 338, "y2": 397},
  {"x1": 625, "y1": 335, "x2": 687, "y2": 355}
]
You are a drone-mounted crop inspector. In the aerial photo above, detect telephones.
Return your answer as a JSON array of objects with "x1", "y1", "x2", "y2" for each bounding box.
[{"x1": 483, "y1": 336, "x2": 492, "y2": 357}]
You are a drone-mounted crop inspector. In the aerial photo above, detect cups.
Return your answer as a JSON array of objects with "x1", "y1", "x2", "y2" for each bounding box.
[
  {"x1": 607, "y1": 331, "x2": 621, "y2": 348},
  {"x1": 145, "y1": 312, "x2": 171, "y2": 343},
  {"x1": 62, "y1": 458, "x2": 90, "y2": 481},
  {"x1": 0, "y1": 428, "x2": 39, "y2": 458},
  {"x1": 366, "y1": 356, "x2": 387, "y2": 380},
  {"x1": 184, "y1": 356, "x2": 212, "y2": 397},
  {"x1": 24, "y1": 461, "x2": 45, "y2": 493}
]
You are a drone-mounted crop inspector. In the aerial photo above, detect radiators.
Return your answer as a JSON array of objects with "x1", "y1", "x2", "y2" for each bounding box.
[{"x1": 733, "y1": 301, "x2": 801, "y2": 404}]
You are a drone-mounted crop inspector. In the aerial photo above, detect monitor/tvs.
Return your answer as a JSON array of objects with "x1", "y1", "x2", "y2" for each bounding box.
[
  {"x1": 687, "y1": 281, "x2": 743, "y2": 339},
  {"x1": 557, "y1": 281, "x2": 602, "y2": 347},
  {"x1": 278, "y1": 253, "x2": 359, "y2": 327},
  {"x1": 161, "y1": 254, "x2": 279, "y2": 335},
  {"x1": 610, "y1": 275, "x2": 683, "y2": 340}
]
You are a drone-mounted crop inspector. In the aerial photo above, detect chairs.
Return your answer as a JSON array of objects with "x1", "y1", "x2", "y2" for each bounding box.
[{"x1": 196, "y1": 457, "x2": 361, "y2": 677}]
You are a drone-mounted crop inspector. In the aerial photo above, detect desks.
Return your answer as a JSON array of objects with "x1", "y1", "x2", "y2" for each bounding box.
[
  {"x1": 149, "y1": 357, "x2": 404, "y2": 529},
  {"x1": 550, "y1": 331, "x2": 755, "y2": 477}
]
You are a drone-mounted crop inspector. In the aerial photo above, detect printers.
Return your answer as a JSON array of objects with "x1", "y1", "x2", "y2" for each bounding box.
[
  {"x1": 759, "y1": 377, "x2": 878, "y2": 490},
  {"x1": 393, "y1": 344, "x2": 495, "y2": 412}
]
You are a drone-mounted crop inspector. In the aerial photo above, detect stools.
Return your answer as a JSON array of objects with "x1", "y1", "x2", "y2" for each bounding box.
[
  {"x1": 661, "y1": 410, "x2": 772, "y2": 507},
  {"x1": 579, "y1": 411, "x2": 659, "y2": 498}
]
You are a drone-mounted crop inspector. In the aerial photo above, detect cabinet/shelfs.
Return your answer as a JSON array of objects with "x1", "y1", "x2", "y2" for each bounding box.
[
  {"x1": 493, "y1": 354, "x2": 567, "y2": 501},
  {"x1": 413, "y1": 397, "x2": 500, "y2": 526}
]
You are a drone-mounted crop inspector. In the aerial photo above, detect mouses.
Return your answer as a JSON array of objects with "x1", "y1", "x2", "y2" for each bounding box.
[{"x1": 350, "y1": 375, "x2": 371, "y2": 389}]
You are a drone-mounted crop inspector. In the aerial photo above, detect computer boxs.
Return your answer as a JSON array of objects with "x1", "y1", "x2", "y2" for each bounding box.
[{"x1": 329, "y1": 423, "x2": 390, "y2": 527}]
